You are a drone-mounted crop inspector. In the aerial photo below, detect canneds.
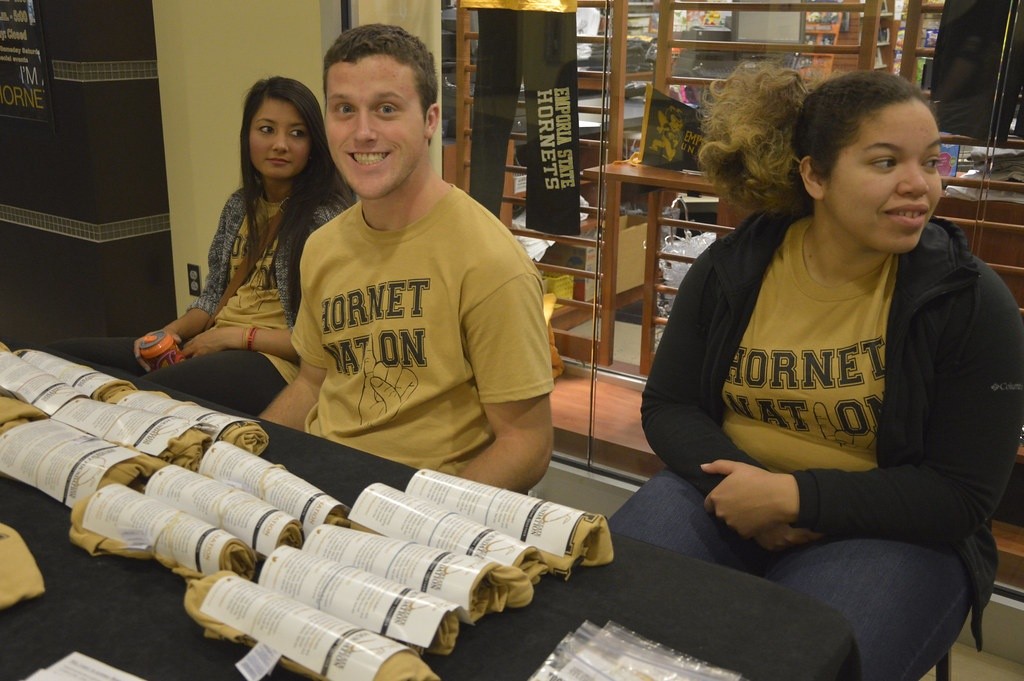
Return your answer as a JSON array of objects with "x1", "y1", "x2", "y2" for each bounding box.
[{"x1": 139, "y1": 331, "x2": 182, "y2": 371}]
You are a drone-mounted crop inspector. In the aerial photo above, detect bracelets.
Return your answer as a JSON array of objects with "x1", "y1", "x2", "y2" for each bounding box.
[
  {"x1": 247, "y1": 328, "x2": 257, "y2": 351},
  {"x1": 242, "y1": 328, "x2": 247, "y2": 350}
]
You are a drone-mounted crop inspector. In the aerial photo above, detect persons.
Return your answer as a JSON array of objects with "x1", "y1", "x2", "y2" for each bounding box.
[
  {"x1": 257, "y1": 23, "x2": 554, "y2": 492},
  {"x1": 31, "y1": 77, "x2": 354, "y2": 419},
  {"x1": 604, "y1": 64, "x2": 1024, "y2": 681}
]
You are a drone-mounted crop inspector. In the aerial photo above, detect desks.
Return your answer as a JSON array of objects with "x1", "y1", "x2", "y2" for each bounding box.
[
  {"x1": 583, "y1": 158, "x2": 1024, "y2": 375},
  {"x1": 0, "y1": 337, "x2": 864, "y2": 681}
]
